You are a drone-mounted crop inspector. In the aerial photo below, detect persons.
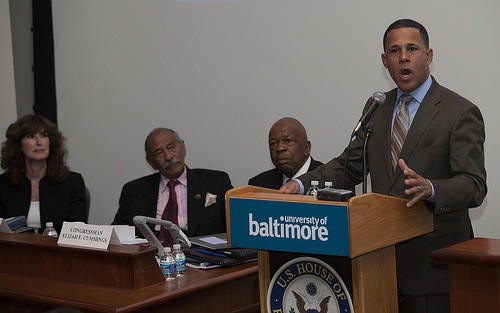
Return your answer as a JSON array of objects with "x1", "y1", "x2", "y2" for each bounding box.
[
  {"x1": 0, "y1": 114, "x2": 88, "y2": 238},
  {"x1": 279, "y1": 19, "x2": 488, "y2": 313},
  {"x1": 248, "y1": 117, "x2": 355, "y2": 200},
  {"x1": 110, "y1": 128, "x2": 234, "y2": 245}
]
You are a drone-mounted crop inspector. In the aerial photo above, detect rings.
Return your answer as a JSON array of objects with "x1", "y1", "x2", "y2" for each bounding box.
[{"x1": 417, "y1": 186, "x2": 422, "y2": 192}]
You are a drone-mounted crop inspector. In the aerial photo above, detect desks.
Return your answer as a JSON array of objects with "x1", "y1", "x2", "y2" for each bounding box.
[{"x1": 0, "y1": 231, "x2": 260, "y2": 313}]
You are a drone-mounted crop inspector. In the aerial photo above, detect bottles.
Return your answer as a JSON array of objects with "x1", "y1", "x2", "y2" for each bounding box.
[
  {"x1": 42, "y1": 222, "x2": 58, "y2": 238},
  {"x1": 324, "y1": 182, "x2": 332, "y2": 189},
  {"x1": 160, "y1": 247, "x2": 177, "y2": 281},
  {"x1": 306, "y1": 180, "x2": 319, "y2": 196},
  {"x1": 172, "y1": 244, "x2": 186, "y2": 278}
]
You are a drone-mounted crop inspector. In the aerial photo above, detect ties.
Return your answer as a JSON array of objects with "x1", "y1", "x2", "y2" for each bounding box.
[
  {"x1": 390, "y1": 94, "x2": 413, "y2": 172},
  {"x1": 159, "y1": 180, "x2": 181, "y2": 247}
]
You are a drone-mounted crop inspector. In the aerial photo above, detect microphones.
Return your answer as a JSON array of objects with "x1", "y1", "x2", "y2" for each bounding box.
[
  {"x1": 362, "y1": 123, "x2": 374, "y2": 193},
  {"x1": 351, "y1": 92, "x2": 386, "y2": 136}
]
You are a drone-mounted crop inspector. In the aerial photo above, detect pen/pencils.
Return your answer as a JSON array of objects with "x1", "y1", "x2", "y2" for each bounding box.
[{"x1": 196, "y1": 250, "x2": 225, "y2": 257}]
